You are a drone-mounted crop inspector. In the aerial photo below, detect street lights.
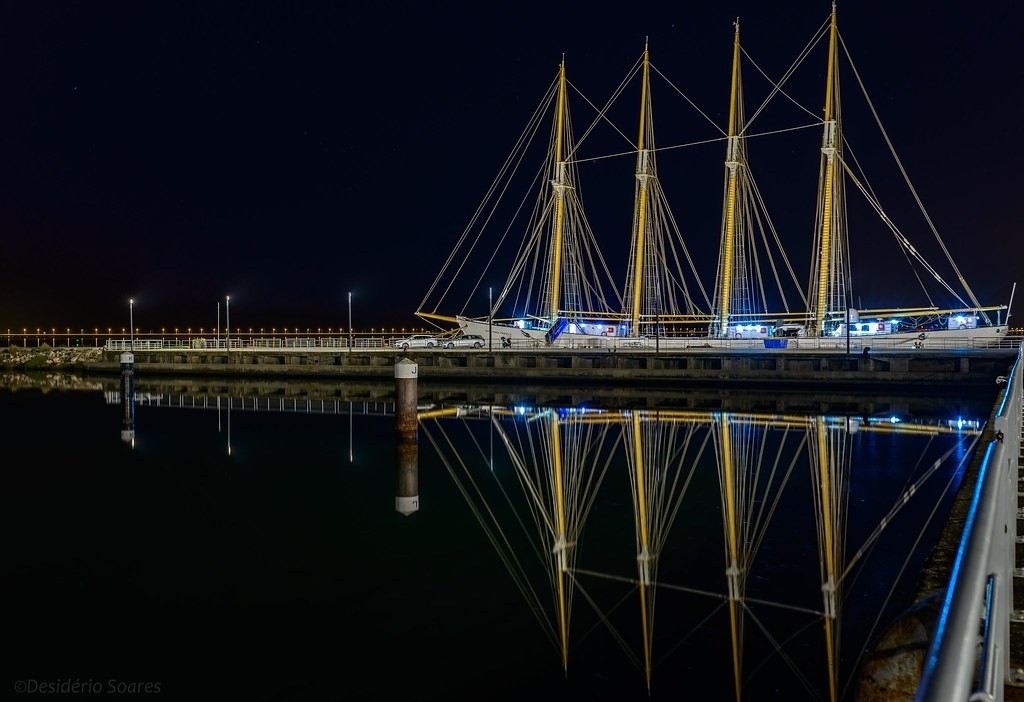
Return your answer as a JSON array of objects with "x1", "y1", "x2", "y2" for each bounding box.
[
  {"x1": 348, "y1": 292, "x2": 353, "y2": 349},
  {"x1": 226, "y1": 295, "x2": 231, "y2": 334},
  {"x1": 130, "y1": 299, "x2": 134, "y2": 350}
]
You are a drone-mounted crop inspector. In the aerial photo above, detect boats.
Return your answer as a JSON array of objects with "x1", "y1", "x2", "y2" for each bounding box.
[
  {"x1": 416, "y1": 408, "x2": 1024, "y2": 700},
  {"x1": 414, "y1": 1, "x2": 1015, "y2": 347}
]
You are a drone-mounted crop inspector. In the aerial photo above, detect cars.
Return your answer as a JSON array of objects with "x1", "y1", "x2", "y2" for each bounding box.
[
  {"x1": 441, "y1": 334, "x2": 486, "y2": 349},
  {"x1": 393, "y1": 334, "x2": 439, "y2": 348}
]
